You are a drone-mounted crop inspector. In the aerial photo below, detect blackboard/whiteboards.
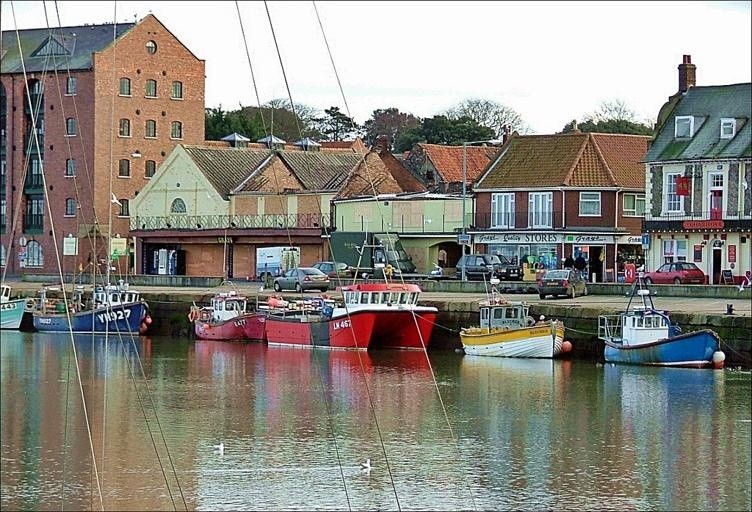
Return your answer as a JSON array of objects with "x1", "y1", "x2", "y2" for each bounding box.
[
  {"x1": 723, "y1": 270, "x2": 734, "y2": 283},
  {"x1": 721, "y1": 270, "x2": 725, "y2": 283}
]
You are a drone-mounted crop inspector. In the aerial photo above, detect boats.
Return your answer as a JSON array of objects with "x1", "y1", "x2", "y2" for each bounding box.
[
  {"x1": 599, "y1": 272, "x2": 720, "y2": 368},
  {"x1": 190, "y1": 282, "x2": 439, "y2": 351},
  {"x1": 0, "y1": 280, "x2": 151, "y2": 336},
  {"x1": 460, "y1": 273, "x2": 564, "y2": 359}
]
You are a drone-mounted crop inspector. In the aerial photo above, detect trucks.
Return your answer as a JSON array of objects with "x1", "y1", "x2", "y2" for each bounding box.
[{"x1": 331, "y1": 231, "x2": 417, "y2": 280}]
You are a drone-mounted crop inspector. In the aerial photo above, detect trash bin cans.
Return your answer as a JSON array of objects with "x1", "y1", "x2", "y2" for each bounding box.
[{"x1": 727, "y1": 304, "x2": 735, "y2": 315}]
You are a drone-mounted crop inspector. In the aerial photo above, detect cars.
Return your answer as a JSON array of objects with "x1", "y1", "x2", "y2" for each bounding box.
[
  {"x1": 538, "y1": 269, "x2": 588, "y2": 299},
  {"x1": 273, "y1": 267, "x2": 331, "y2": 293},
  {"x1": 311, "y1": 262, "x2": 353, "y2": 285},
  {"x1": 643, "y1": 262, "x2": 705, "y2": 284}
]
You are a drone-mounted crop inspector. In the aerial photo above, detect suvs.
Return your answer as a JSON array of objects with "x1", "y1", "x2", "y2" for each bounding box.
[{"x1": 456, "y1": 254, "x2": 523, "y2": 281}]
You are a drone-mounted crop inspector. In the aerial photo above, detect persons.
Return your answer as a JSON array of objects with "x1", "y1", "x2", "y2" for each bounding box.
[
  {"x1": 574, "y1": 253, "x2": 586, "y2": 281},
  {"x1": 438, "y1": 246, "x2": 447, "y2": 268},
  {"x1": 564, "y1": 253, "x2": 575, "y2": 270}
]
